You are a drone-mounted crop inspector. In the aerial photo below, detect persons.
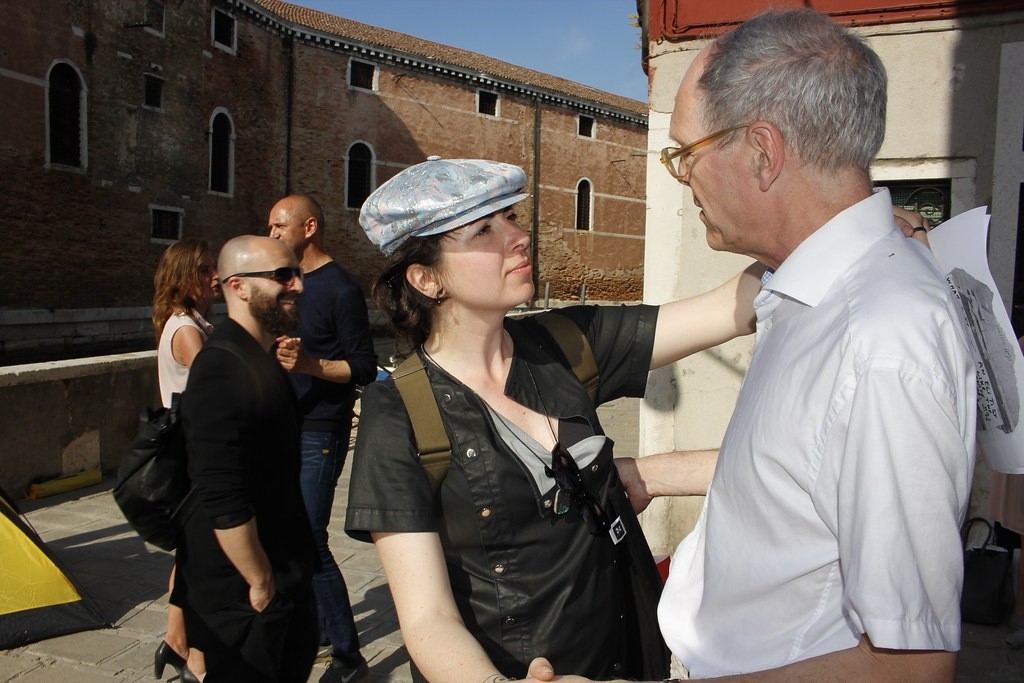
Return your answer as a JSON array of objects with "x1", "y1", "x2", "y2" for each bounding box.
[
  {"x1": 182, "y1": 235, "x2": 320, "y2": 682},
  {"x1": 269, "y1": 193, "x2": 378, "y2": 683},
  {"x1": 153, "y1": 237, "x2": 225, "y2": 679},
  {"x1": 513, "y1": 8, "x2": 978, "y2": 683},
  {"x1": 344, "y1": 154, "x2": 932, "y2": 683}
]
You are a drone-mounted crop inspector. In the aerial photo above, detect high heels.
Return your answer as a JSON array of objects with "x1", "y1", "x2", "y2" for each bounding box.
[{"x1": 154, "y1": 640, "x2": 201, "y2": 683}]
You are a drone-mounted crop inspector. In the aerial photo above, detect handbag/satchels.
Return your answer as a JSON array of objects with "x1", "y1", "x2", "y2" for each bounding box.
[{"x1": 960, "y1": 517, "x2": 1015, "y2": 628}]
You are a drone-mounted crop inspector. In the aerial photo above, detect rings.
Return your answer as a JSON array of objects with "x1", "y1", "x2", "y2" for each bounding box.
[{"x1": 913, "y1": 227, "x2": 927, "y2": 233}]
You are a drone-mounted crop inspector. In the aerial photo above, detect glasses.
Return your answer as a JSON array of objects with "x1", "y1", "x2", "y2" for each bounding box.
[
  {"x1": 222, "y1": 266, "x2": 302, "y2": 286},
  {"x1": 552, "y1": 441, "x2": 612, "y2": 538},
  {"x1": 660, "y1": 123, "x2": 752, "y2": 186}
]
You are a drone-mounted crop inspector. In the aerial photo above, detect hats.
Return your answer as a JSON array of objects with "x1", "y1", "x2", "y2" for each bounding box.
[{"x1": 358, "y1": 155, "x2": 530, "y2": 259}]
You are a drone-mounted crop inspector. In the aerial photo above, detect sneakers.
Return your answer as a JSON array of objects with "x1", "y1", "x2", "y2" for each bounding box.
[{"x1": 319, "y1": 657, "x2": 369, "y2": 683}]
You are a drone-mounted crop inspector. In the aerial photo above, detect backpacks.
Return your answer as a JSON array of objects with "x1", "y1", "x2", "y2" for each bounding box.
[{"x1": 113, "y1": 336, "x2": 279, "y2": 552}]
